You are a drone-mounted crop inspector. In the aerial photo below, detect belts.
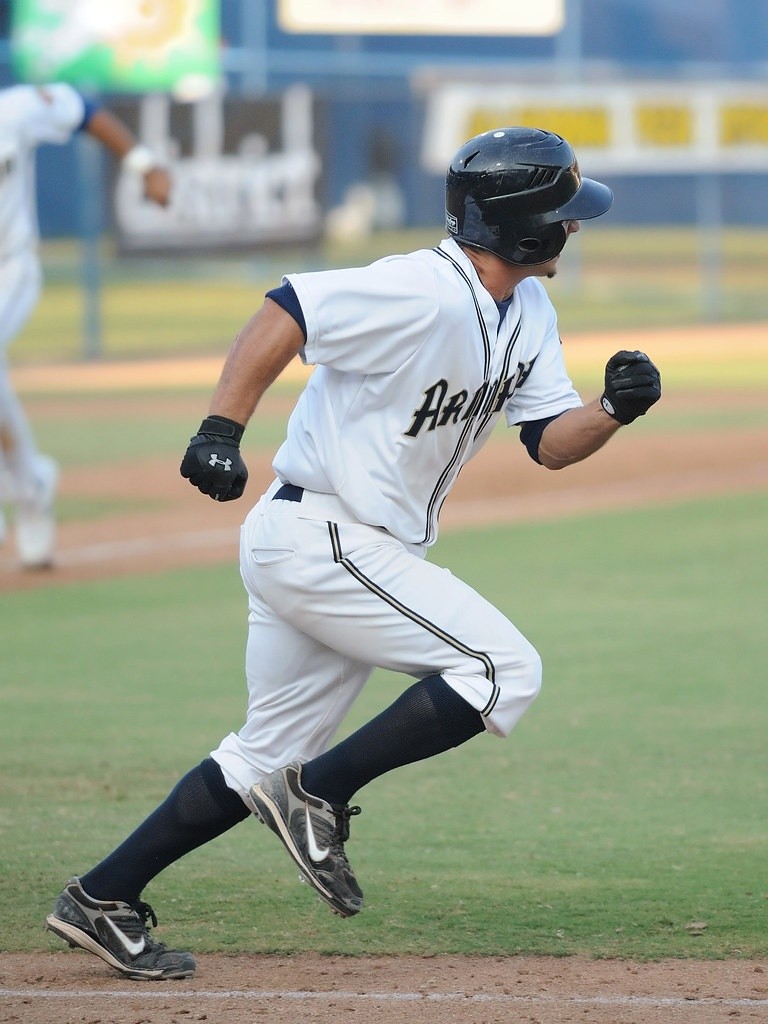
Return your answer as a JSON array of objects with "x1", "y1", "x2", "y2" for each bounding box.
[{"x1": 272, "y1": 483, "x2": 304, "y2": 502}]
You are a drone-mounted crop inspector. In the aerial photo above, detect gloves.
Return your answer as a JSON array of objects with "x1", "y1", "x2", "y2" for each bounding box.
[
  {"x1": 180, "y1": 415, "x2": 248, "y2": 502},
  {"x1": 600, "y1": 350, "x2": 662, "y2": 425}
]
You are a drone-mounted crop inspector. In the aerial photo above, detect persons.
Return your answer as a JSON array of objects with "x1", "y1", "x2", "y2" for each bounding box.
[
  {"x1": 45, "y1": 128, "x2": 660, "y2": 982},
  {"x1": 0, "y1": 84, "x2": 176, "y2": 570}
]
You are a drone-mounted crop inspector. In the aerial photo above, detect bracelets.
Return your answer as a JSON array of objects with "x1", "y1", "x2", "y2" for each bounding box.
[{"x1": 121, "y1": 145, "x2": 154, "y2": 176}]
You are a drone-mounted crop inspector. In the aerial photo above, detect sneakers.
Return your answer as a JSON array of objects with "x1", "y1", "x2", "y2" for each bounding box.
[
  {"x1": 249, "y1": 760, "x2": 363, "y2": 918},
  {"x1": 46, "y1": 876, "x2": 196, "y2": 981}
]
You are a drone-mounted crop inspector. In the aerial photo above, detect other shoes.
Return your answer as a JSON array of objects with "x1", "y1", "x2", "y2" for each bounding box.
[{"x1": 16, "y1": 458, "x2": 56, "y2": 566}]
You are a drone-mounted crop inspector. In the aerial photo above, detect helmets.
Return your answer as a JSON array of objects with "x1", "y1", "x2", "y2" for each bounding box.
[{"x1": 445, "y1": 127, "x2": 614, "y2": 267}]
